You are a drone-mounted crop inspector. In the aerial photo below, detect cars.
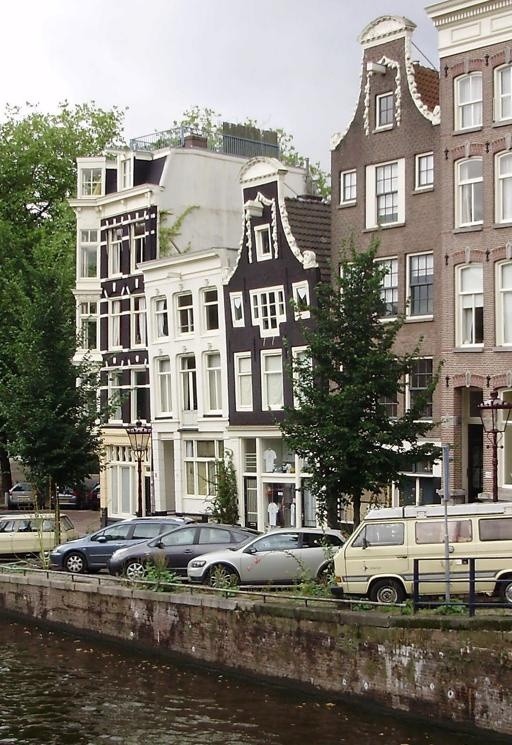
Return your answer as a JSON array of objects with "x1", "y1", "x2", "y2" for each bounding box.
[
  {"x1": 48, "y1": 517, "x2": 195, "y2": 574},
  {"x1": 8, "y1": 480, "x2": 101, "y2": 508},
  {"x1": 108, "y1": 519, "x2": 257, "y2": 578},
  {"x1": 0, "y1": 513, "x2": 81, "y2": 555},
  {"x1": 186, "y1": 527, "x2": 351, "y2": 592}
]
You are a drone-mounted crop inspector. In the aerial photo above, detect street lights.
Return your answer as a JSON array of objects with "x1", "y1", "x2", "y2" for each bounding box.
[
  {"x1": 478, "y1": 388, "x2": 512, "y2": 503},
  {"x1": 127, "y1": 422, "x2": 151, "y2": 516}
]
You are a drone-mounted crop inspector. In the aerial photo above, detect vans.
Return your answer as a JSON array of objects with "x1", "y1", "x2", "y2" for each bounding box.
[{"x1": 322, "y1": 504, "x2": 512, "y2": 605}]
